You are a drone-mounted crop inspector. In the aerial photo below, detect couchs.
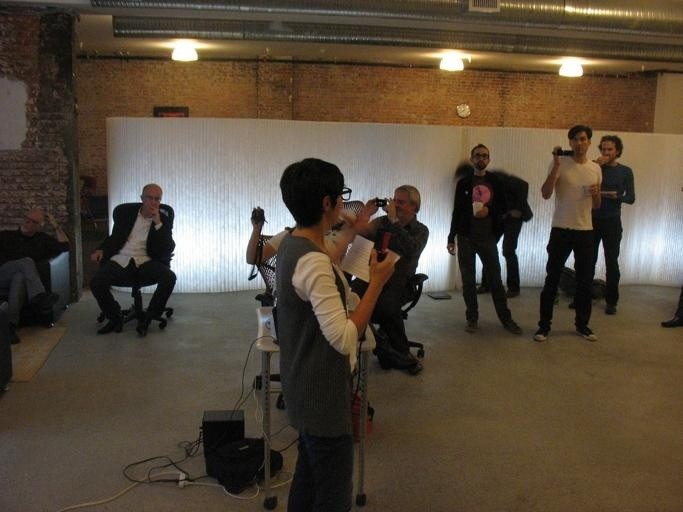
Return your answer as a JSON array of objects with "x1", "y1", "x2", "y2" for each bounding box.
[
  {"x1": 2, "y1": 235, "x2": 72, "y2": 331},
  {"x1": 0, "y1": 330, "x2": 16, "y2": 397}
]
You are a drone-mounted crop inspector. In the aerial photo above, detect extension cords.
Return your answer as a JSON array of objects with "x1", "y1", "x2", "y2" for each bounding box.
[{"x1": 179, "y1": 472, "x2": 185, "y2": 488}]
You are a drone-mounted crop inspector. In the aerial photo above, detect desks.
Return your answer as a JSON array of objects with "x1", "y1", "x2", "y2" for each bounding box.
[{"x1": 250, "y1": 304, "x2": 381, "y2": 512}]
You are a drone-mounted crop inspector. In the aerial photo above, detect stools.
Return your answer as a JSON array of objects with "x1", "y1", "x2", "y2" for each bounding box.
[{"x1": 353, "y1": 270, "x2": 428, "y2": 362}]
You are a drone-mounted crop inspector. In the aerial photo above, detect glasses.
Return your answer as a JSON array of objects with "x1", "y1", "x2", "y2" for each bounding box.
[
  {"x1": 334, "y1": 188, "x2": 352, "y2": 200},
  {"x1": 471, "y1": 152, "x2": 488, "y2": 160}
]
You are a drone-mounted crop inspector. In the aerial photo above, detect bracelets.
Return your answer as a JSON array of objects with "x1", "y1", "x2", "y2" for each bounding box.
[{"x1": 53, "y1": 225, "x2": 60, "y2": 231}]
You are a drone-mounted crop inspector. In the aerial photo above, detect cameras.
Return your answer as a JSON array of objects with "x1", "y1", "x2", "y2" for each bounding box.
[
  {"x1": 376, "y1": 199, "x2": 387, "y2": 207},
  {"x1": 252, "y1": 208, "x2": 264, "y2": 224},
  {"x1": 557, "y1": 149, "x2": 574, "y2": 156}
]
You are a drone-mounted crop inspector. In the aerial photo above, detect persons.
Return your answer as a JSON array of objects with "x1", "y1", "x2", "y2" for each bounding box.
[
  {"x1": 447, "y1": 146, "x2": 523, "y2": 333},
  {"x1": 455, "y1": 165, "x2": 532, "y2": 298},
  {"x1": 568, "y1": 135, "x2": 634, "y2": 314},
  {"x1": 533, "y1": 125, "x2": 603, "y2": 342},
  {"x1": 356, "y1": 185, "x2": 427, "y2": 373},
  {"x1": 89, "y1": 184, "x2": 177, "y2": 336},
  {"x1": 661, "y1": 285, "x2": 682, "y2": 327},
  {"x1": 277, "y1": 158, "x2": 396, "y2": 512},
  {"x1": 0, "y1": 210, "x2": 71, "y2": 344},
  {"x1": 248, "y1": 207, "x2": 355, "y2": 409}
]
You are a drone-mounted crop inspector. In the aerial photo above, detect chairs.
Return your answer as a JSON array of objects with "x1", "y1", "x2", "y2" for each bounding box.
[{"x1": 95, "y1": 201, "x2": 175, "y2": 331}]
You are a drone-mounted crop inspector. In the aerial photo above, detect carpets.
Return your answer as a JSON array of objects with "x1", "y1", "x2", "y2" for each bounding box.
[{"x1": 0, "y1": 319, "x2": 66, "y2": 385}]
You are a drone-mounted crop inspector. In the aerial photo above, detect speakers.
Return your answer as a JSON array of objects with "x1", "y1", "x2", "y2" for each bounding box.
[{"x1": 202, "y1": 409, "x2": 244, "y2": 479}]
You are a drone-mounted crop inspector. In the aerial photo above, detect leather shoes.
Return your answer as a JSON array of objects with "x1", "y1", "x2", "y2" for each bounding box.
[
  {"x1": 380, "y1": 352, "x2": 422, "y2": 374},
  {"x1": 136, "y1": 315, "x2": 150, "y2": 337},
  {"x1": 96, "y1": 319, "x2": 123, "y2": 335}
]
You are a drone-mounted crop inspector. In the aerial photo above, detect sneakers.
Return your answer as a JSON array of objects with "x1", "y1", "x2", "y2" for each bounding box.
[
  {"x1": 533, "y1": 328, "x2": 549, "y2": 341},
  {"x1": 505, "y1": 321, "x2": 521, "y2": 335},
  {"x1": 662, "y1": 315, "x2": 683, "y2": 328},
  {"x1": 506, "y1": 289, "x2": 520, "y2": 298},
  {"x1": 577, "y1": 327, "x2": 596, "y2": 341},
  {"x1": 477, "y1": 286, "x2": 489, "y2": 294},
  {"x1": 467, "y1": 319, "x2": 478, "y2": 333},
  {"x1": 39, "y1": 294, "x2": 58, "y2": 317},
  {"x1": 606, "y1": 307, "x2": 618, "y2": 315}
]
